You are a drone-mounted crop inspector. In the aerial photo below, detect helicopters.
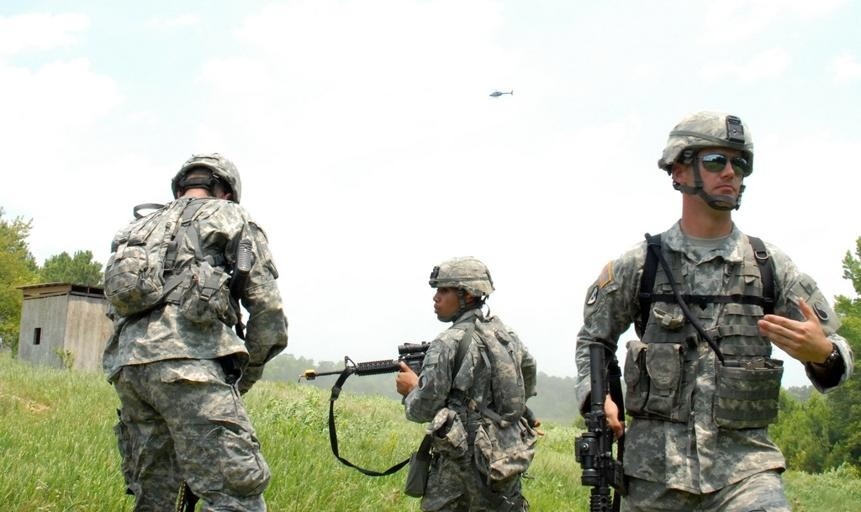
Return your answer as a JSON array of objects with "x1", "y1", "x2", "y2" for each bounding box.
[{"x1": 488, "y1": 89, "x2": 514, "y2": 97}]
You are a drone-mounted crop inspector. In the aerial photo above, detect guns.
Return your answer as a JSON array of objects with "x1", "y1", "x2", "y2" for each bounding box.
[
  {"x1": 299, "y1": 342, "x2": 431, "y2": 380},
  {"x1": 576, "y1": 342, "x2": 629, "y2": 511},
  {"x1": 175, "y1": 367, "x2": 242, "y2": 512}
]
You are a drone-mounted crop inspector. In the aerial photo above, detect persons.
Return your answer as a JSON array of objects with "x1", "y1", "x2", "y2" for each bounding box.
[
  {"x1": 394, "y1": 259, "x2": 544, "y2": 512},
  {"x1": 102, "y1": 151, "x2": 287, "y2": 512},
  {"x1": 574, "y1": 112, "x2": 854, "y2": 512}
]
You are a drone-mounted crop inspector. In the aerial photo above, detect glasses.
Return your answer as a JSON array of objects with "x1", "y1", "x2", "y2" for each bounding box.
[{"x1": 695, "y1": 151, "x2": 750, "y2": 177}]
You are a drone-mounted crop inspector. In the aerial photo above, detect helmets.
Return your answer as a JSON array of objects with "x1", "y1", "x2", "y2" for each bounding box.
[
  {"x1": 171, "y1": 152, "x2": 241, "y2": 203},
  {"x1": 429, "y1": 258, "x2": 494, "y2": 296},
  {"x1": 662, "y1": 111, "x2": 754, "y2": 177}
]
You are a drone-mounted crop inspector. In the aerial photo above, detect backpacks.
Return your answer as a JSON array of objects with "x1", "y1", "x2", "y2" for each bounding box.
[{"x1": 102, "y1": 196, "x2": 190, "y2": 319}]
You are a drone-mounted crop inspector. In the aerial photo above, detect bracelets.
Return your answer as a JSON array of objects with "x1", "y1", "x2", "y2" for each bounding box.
[{"x1": 813, "y1": 341, "x2": 840, "y2": 371}]
